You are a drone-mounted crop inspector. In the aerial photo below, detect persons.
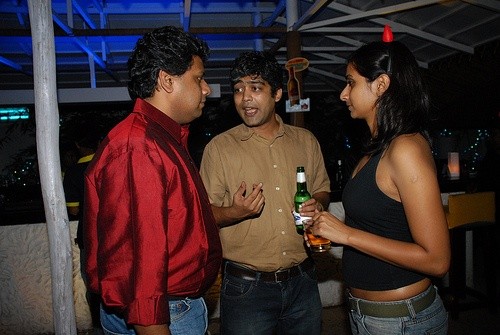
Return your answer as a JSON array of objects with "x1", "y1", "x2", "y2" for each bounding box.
[
  {"x1": 199, "y1": 50, "x2": 333, "y2": 335},
  {"x1": 79, "y1": 26, "x2": 223, "y2": 335},
  {"x1": 309, "y1": 41, "x2": 453, "y2": 335},
  {"x1": 64, "y1": 125, "x2": 98, "y2": 217}
]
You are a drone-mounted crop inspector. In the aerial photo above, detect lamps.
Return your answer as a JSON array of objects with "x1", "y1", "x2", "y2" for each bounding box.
[{"x1": 448, "y1": 152, "x2": 459, "y2": 180}]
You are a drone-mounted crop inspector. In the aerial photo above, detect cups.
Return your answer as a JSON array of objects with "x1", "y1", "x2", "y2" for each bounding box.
[{"x1": 302, "y1": 220, "x2": 331, "y2": 253}]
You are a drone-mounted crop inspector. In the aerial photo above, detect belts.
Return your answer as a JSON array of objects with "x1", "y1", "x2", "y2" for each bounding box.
[
  {"x1": 347, "y1": 286, "x2": 436, "y2": 318},
  {"x1": 226, "y1": 259, "x2": 312, "y2": 281}
]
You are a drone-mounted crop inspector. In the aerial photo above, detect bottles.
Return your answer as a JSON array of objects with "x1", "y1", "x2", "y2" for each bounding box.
[{"x1": 294, "y1": 166, "x2": 312, "y2": 235}]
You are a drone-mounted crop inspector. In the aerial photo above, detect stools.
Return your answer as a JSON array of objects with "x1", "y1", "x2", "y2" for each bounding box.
[{"x1": 443, "y1": 191, "x2": 500, "y2": 292}]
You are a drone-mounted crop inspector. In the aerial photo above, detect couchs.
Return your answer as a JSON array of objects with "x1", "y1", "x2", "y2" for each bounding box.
[{"x1": 0, "y1": 221, "x2": 221, "y2": 335}]
